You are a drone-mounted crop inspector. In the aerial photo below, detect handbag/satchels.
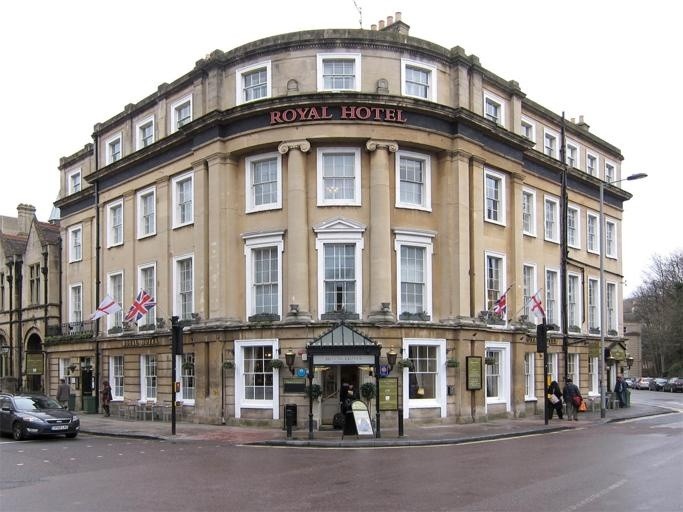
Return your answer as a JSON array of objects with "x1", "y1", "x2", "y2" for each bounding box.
[{"x1": 572, "y1": 396, "x2": 588, "y2": 412}]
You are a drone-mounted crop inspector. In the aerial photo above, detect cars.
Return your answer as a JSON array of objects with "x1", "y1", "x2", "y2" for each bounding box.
[
  {"x1": 0, "y1": 394, "x2": 80, "y2": 441},
  {"x1": 624, "y1": 376, "x2": 683, "y2": 392}
]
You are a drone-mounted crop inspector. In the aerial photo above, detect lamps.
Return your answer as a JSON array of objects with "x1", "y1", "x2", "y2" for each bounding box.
[
  {"x1": 285, "y1": 349, "x2": 295, "y2": 375},
  {"x1": 381, "y1": 303, "x2": 390, "y2": 312},
  {"x1": 387, "y1": 344, "x2": 398, "y2": 372},
  {"x1": 290, "y1": 304, "x2": 299, "y2": 313},
  {"x1": 624, "y1": 353, "x2": 634, "y2": 368},
  {"x1": 606, "y1": 353, "x2": 615, "y2": 371}
]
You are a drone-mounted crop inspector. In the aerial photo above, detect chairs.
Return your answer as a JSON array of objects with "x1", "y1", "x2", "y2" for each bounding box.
[{"x1": 107, "y1": 399, "x2": 196, "y2": 423}]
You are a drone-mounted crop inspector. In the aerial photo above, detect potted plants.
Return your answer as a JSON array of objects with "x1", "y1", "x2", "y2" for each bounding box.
[
  {"x1": 446, "y1": 358, "x2": 460, "y2": 368},
  {"x1": 485, "y1": 357, "x2": 496, "y2": 365},
  {"x1": 305, "y1": 383, "x2": 321, "y2": 400},
  {"x1": 359, "y1": 382, "x2": 377, "y2": 399}
]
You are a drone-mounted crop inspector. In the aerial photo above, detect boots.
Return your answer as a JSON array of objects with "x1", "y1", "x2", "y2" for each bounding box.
[{"x1": 102, "y1": 404, "x2": 110, "y2": 417}]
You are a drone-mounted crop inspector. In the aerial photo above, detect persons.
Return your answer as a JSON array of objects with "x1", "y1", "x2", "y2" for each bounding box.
[
  {"x1": 614, "y1": 373, "x2": 628, "y2": 408},
  {"x1": 547, "y1": 379, "x2": 583, "y2": 421},
  {"x1": 99, "y1": 381, "x2": 112, "y2": 417},
  {"x1": 56, "y1": 378, "x2": 71, "y2": 411}
]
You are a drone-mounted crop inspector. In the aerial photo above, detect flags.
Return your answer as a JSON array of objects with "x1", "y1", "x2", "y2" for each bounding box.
[
  {"x1": 526, "y1": 292, "x2": 545, "y2": 325},
  {"x1": 124, "y1": 290, "x2": 158, "y2": 322},
  {"x1": 493, "y1": 294, "x2": 507, "y2": 321},
  {"x1": 88, "y1": 295, "x2": 122, "y2": 321}
]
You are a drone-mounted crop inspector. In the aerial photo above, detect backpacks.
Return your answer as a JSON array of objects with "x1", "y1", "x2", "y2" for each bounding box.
[{"x1": 547, "y1": 394, "x2": 559, "y2": 404}]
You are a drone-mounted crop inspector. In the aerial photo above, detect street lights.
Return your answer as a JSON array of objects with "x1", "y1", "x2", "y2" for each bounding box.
[{"x1": 599, "y1": 168, "x2": 649, "y2": 419}]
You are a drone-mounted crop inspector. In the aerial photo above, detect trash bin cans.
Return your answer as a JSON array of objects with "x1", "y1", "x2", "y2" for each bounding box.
[
  {"x1": 83, "y1": 396, "x2": 97, "y2": 414},
  {"x1": 605, "y1": 392, "x2": 618, "y2": 409},
  {"x1": 283, "y1": 404, "x2": 298, "y2": 431},
  {"x1": 68, "y1": 394, "x2": 75, "y2": 411}
]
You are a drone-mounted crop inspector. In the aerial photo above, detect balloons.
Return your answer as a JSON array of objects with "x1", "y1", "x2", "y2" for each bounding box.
[{"x1": 297, "y1": 368, "x2": 306, "y2": 377}]
